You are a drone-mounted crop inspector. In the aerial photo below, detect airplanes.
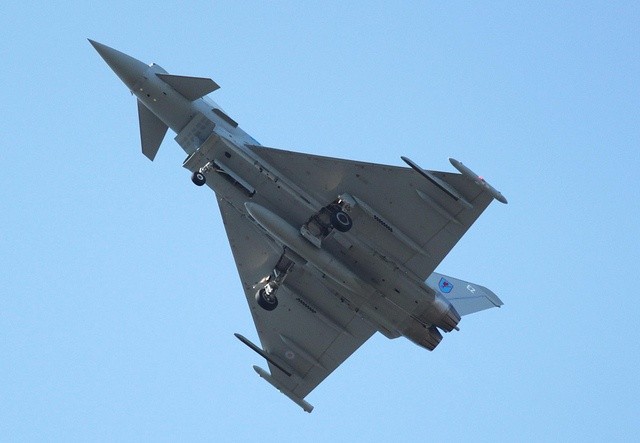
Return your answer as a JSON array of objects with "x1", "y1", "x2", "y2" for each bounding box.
[{"x1": 89, "y1": 38, "x2": 509, "y2": 414}]
